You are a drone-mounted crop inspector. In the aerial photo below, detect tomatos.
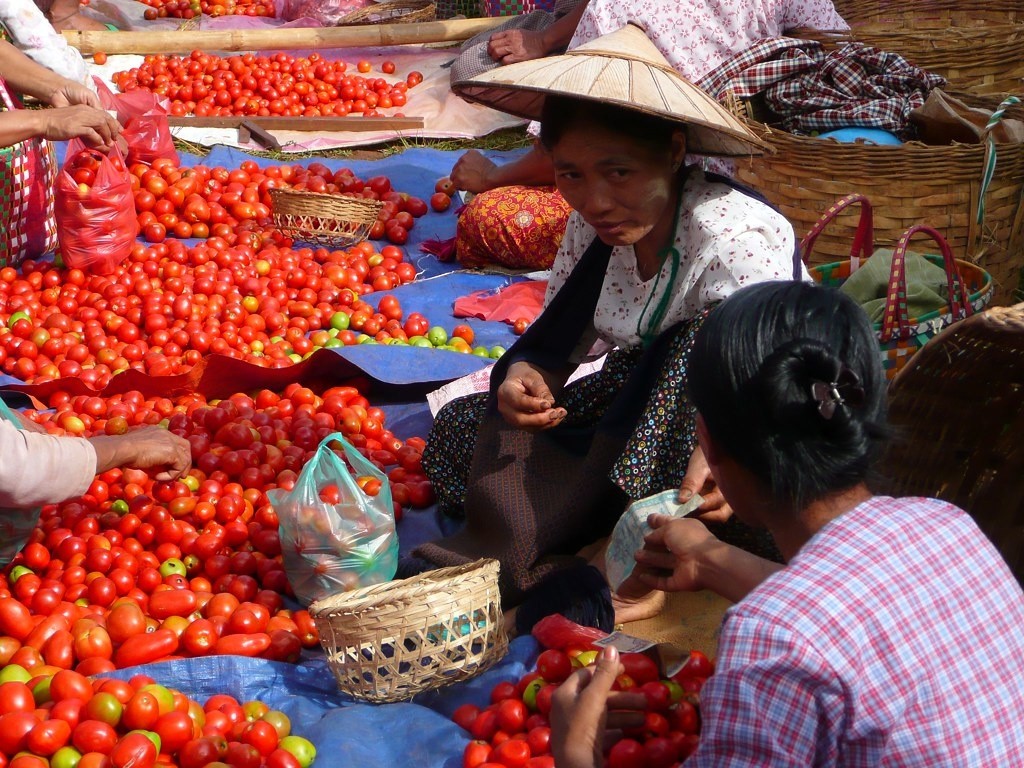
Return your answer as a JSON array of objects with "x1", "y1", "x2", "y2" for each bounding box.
[
  {"x1": 0, "y1": 134, "x2": 724, "y2": 768},
  {"x1": 75, "y1": 0, "x2": 423, "y2": 120}
]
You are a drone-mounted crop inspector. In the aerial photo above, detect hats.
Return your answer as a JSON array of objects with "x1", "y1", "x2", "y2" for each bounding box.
[{"x1": 450, "y1": 18, "x2": 778, "y2": 158}]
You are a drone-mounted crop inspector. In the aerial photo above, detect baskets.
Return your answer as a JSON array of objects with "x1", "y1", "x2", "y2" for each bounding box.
[
  {"x1": 782, "y1": 0, "x2": 1024, "y2": 92},
  {"x1": 712, "y1": 88, "x2": 1024, "y2": 308},
  {"x1": 798, "y1": 192, "x2": 995, "y2": 383},
  {"x1": 484, "y1": 0, "x2": 556, "y2": 17},
  {"x1": 266, "y1": 187, "x2": 387, "y2": 248},
  {"x1": 867, "y1": 302, "x2": 1024, "y2": 589},
  {"x1": 337, "y1": 0, "x2": 438, "y2": 26},
  {"x1": 308, "y1": 557, "x2": 509, "y2": 703},
  {"x1": 0, "y1": 76, "x2": 60, "y2": 268}
]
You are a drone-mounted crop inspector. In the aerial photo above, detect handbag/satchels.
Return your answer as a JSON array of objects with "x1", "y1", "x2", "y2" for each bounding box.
[
  {"x1": 265, "y1": 431, "x2": 401, "y2": 607},
  {"x1": 91, "y1": 73, "x2": 181, "y2": 168},
  {"x1": 53, "y1": 136, "x2": 138, "y2": 276}
]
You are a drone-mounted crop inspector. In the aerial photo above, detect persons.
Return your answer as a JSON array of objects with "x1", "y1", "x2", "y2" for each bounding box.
[
  {"x1": 1, "y1": 397, "x2": 192, "y2": 569},
  {"x1": 0, "y1": 0, "x2": 128, "y2": 162},
  {"x1": 450, "y1": 0, "x2": 852, "y2": 270},
  {"x1": 551, "y1": 280, "x2": 1024, "y2": 768},
  {"x1": 420, "y1": 15, "x2": 814, "y2": 643}
]
